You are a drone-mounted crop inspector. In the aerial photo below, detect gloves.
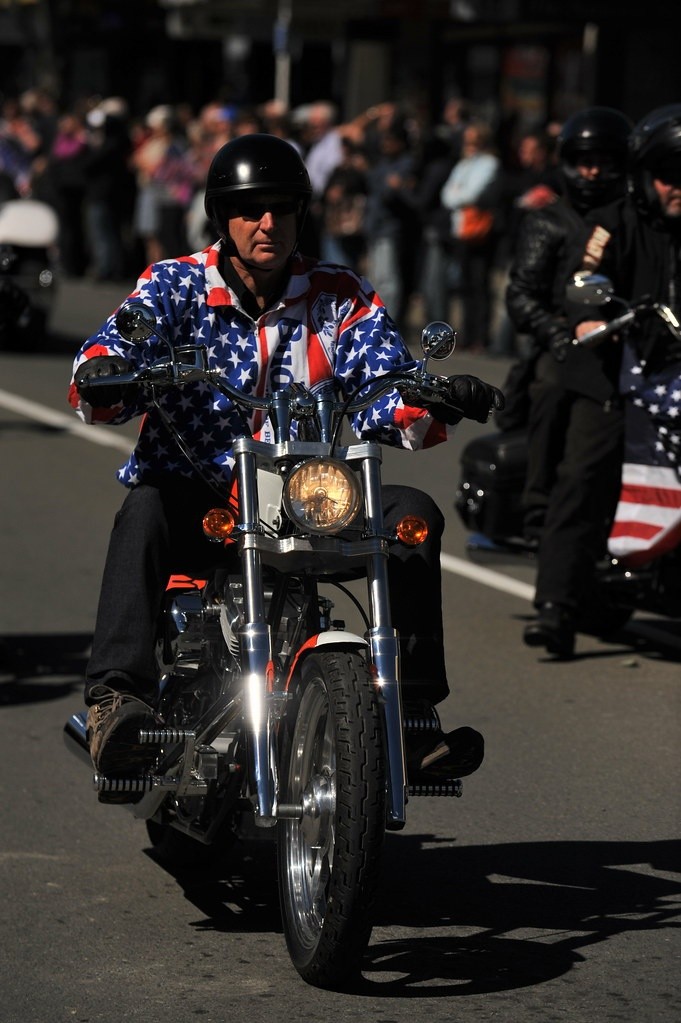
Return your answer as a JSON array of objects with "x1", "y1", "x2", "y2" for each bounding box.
[
  {"x1": 74, "y1": 355, "x2": 140, "y2": 409},
  {"x1": 543, "y1": 321, "x2": 580, "y2": 362},
  {"x1": 427, "y1": 374, "x2": 507, "y2": 426}
]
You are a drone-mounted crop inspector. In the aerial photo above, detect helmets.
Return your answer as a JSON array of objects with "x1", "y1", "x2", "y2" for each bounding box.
[
  {"x1": 548, "y1": 106, "x2": 636, "y2": 206},
  {"x1": 205, "y1": 134, "x2": 312, "y2": 221},
  {"x1": 627, "y1": 101, "x2": 681, "y2": 177}
]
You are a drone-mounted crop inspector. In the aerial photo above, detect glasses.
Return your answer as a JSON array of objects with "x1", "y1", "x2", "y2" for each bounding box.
[{"x1": 223, "y1": 200, "x2": 298, "y2": 222}]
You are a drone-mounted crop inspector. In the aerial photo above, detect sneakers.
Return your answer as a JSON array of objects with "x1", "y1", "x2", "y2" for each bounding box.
[
  {"x1": 405, "y1": 723, "x2": 484, "y2": 787},
  {"x1": 84, "y1": 684, "x2": 166, "y2": 805}
]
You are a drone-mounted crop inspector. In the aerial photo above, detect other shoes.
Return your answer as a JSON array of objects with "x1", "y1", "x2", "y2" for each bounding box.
[
  {"x1": 522, "y1": 509, "x2": 545, "y2": 540},
  {"x1": 456, "y1": 335, "x2": 492, "y2": 353}
]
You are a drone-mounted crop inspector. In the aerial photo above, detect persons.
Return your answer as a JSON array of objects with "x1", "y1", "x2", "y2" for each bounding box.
[
  {"x1": 505, "y1": 102, "x2": 681, "y2": 662},
  {"x1": 0, "y1": 87, "x2": 565, "y2": 354},
  {"x1": 68, "y1": 133, "x2": 496, "y2": 803}
]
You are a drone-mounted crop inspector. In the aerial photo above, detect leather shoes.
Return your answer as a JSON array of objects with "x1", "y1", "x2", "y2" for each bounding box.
[{"x1": 523, "y1": 600, "x2": 576, "y2": 659}]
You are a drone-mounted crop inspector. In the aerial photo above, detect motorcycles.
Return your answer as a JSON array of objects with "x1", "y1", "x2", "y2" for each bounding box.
[
  {"x1": 76, "y1": 304, "x2": 493, "y2": 989},
  {"x1": 455, "y1": 269, "x2": 681, "y2": 612}
]
